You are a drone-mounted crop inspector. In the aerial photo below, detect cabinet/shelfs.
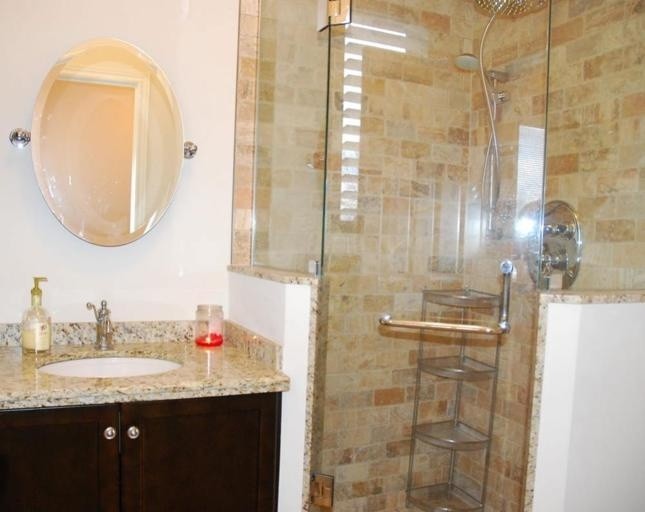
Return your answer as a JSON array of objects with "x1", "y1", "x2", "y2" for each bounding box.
[
  {"x1": 404, "y1": 288, "x2": 503, "y2": 512},
  {"x1": 0, "y1": 392, "x2": 282, "y2": 512}
]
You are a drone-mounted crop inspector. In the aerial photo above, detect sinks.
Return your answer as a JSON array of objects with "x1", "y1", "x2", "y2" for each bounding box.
[{"x1": 35, "y1": 351, "x2": 184, "y2": 376}]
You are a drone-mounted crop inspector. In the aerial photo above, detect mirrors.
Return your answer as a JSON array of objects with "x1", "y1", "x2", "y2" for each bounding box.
[{"x1": 31, "y1": 35, "x2": 185, "y2": 248}]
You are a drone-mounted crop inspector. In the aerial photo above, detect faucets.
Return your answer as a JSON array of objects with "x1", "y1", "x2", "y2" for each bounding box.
[{"x1": 86, "y1": 299, "x2": 115, "y2": 351}]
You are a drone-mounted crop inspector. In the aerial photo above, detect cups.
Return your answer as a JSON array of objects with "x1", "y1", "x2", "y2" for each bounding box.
[{"x1": 195, "y1": 304, "x2": 224, "y2": 347}]
